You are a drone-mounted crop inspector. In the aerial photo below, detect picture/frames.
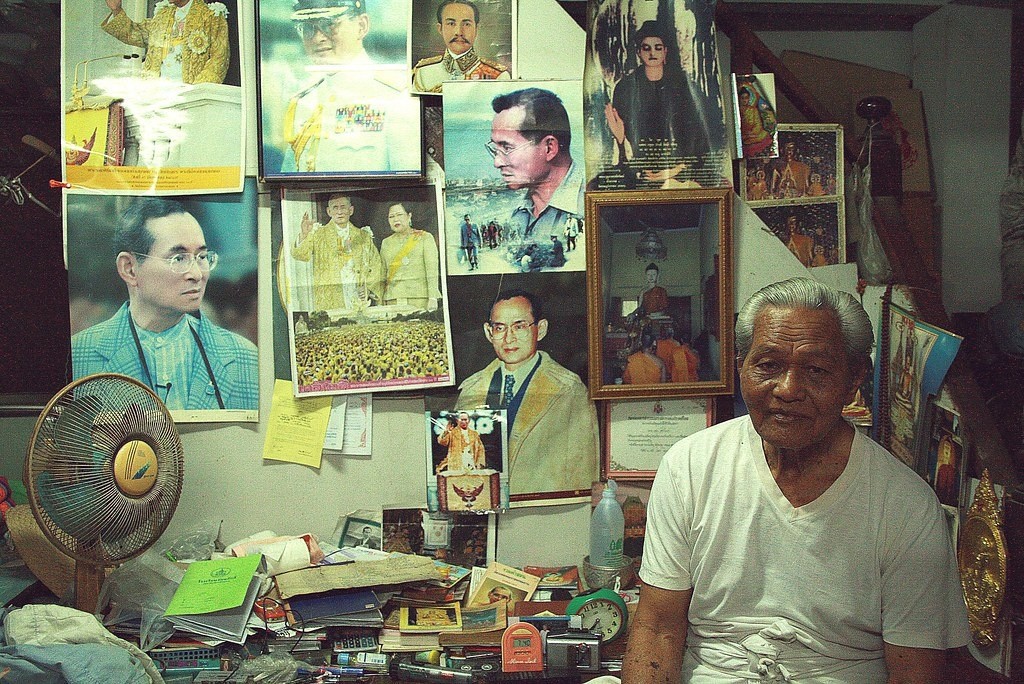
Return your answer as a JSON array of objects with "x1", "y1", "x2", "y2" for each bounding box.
[
  {"x1": 739, "y1": 124, "x2": 847, "y2": 267},
  {"x1": 254, "y1": 0, "x2": 427, "y2": 183},
  {"x1": 583, "y1": 187, "x2": 736, "y2": 401}
]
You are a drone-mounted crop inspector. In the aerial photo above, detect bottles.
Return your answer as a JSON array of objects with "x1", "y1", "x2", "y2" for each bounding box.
[{"x1": 591, "y1": 480, "x2": 625, "y2": 567}]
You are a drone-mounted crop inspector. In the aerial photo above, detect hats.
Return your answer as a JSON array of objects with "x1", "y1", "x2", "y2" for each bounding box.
[
  {"x1": 291, "y1": 0, "x2": 366, "y2": 22},
  {"x1": 551, "y1": 236, "x2": 557, "y2": 240}
]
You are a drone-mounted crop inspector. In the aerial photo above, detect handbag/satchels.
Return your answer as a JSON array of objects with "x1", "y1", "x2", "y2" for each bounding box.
[{"x1": 457, "y1": 249, "x2": 469, "y2": 265}]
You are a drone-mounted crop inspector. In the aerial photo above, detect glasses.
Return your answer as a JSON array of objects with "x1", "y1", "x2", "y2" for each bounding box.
[
  {"x1": 130, "y1": 250, "x2": 219, "y2": 274},
  {"x1": 485, "y1": 139, "x2": 536, "y2": 165},
  {"x1": 295, "y1": 13, "x2": 353, "y2": 40},
  {"x1": 487, "y1": 321, "x2": 536, "y2": 340},
  {"x1": 465, "y1": 219, "x2": 469, "y2": 221}
]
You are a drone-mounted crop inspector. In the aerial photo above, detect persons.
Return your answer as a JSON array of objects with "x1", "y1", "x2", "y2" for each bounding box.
[
  {"x1": 354, "y1": 525, "x2": 377, "y2": 550},
  {"x1": 70, "y1": 1, "x2": 721, "y2": 498},
  {"x1": 482, "y1": 585, "x2": 514, "y2": 617},
  {"x1": 736, "y1": 84, "x2": 829, "y2": 267},
  {"x1": 619, "y1": 279, "x2": 975, "y2": 684}
]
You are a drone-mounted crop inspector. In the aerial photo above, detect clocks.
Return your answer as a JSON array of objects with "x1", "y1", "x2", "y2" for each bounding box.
[{"x1": 564, "y1": 587, "x2": 628, "y2": 647}]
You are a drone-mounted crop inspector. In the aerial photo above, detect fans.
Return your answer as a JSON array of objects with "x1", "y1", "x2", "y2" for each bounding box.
[{"x1": 21, "y1": 372, "x2": 185, "y2": 616}]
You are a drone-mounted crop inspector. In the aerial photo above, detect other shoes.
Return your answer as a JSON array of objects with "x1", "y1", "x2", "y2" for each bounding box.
[
  {"x1": 475, "y1": 263, "x2": 479, "y2": 269},
  {"x1": 470, "y1": 263, "x2": 474, "y2": 270}
]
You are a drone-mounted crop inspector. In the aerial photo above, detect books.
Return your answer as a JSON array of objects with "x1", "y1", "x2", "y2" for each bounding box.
[{"x1": 159, "y1": 506, "x2": 595, "y2": 683}]
